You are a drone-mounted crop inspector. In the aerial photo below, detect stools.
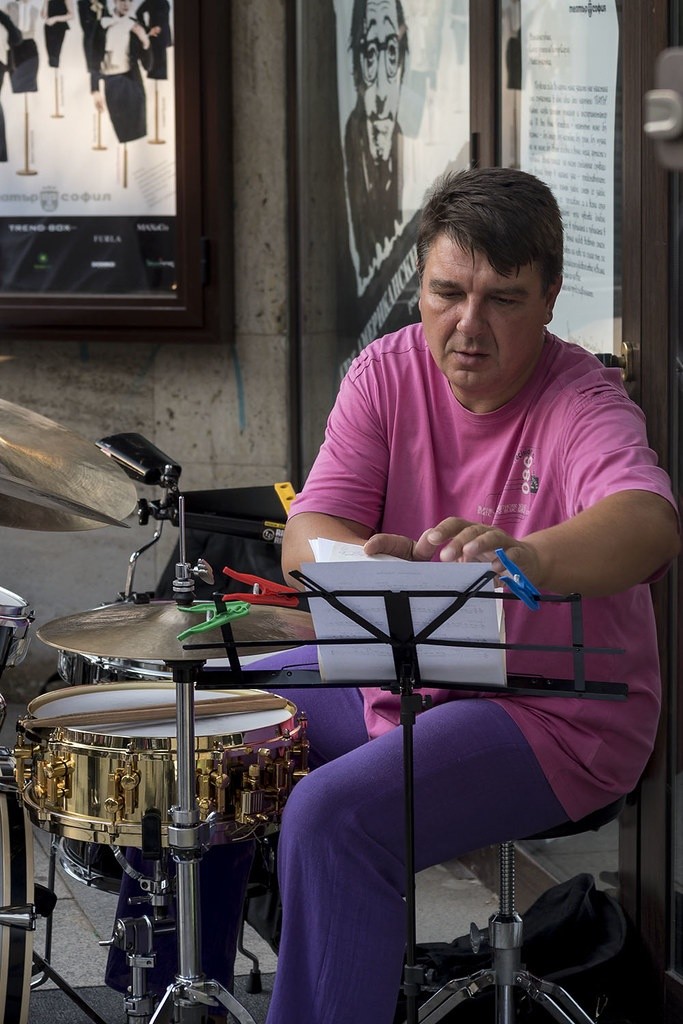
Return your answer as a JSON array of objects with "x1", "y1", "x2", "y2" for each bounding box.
[{"x1": 406, "y1": 784, "x2": 598, "y2": 1022}]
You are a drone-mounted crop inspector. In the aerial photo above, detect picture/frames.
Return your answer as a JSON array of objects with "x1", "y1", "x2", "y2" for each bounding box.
[{"x1": 0, "y1": 2, "x2": 235, "y2": 351}]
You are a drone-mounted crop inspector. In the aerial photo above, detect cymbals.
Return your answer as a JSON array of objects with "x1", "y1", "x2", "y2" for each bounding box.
[
  {"x1": 1, "y1": 397, "x2": 138, "y2": 532},
  {"x1": 33, "y1": 599, "x2": 318, "y2": 660}
]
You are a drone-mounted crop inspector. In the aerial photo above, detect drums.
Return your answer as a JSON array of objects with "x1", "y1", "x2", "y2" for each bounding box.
[
  {"x1": 57, "y1": 647, "x2": 172, "y2": 687},
  {"x1": 59, "y1": 837, "x2": 177, "y2": 894},
  {"x1": 12, "y1": 678, "x2": 311, "y2": 847}
]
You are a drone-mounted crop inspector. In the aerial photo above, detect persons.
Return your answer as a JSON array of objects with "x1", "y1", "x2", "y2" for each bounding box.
[
  {"x1": 90, "y1": 1, "x2": 152, "y2": 144},
  {"x1": 102, "y1": 169, "x2": 678, "y2": 1021},
  {"x1": 8, "y1": 0, "x2": 42, "y2": 93},
  {"x1": 76, "y1": 1, "x2": 110, "y2": 73},
  {"x1": 135, "y1": 1, "x2": 172, "y2": 81},
  {"x1": 40, "y1": 0, "x2": 71, "y2": 67},
  {"x1": 343, "y1": 0, "x2": 409, "y2": 282},
  {"x1": 0, "y1": 9, "x2": 25, "y2": 163}
]
